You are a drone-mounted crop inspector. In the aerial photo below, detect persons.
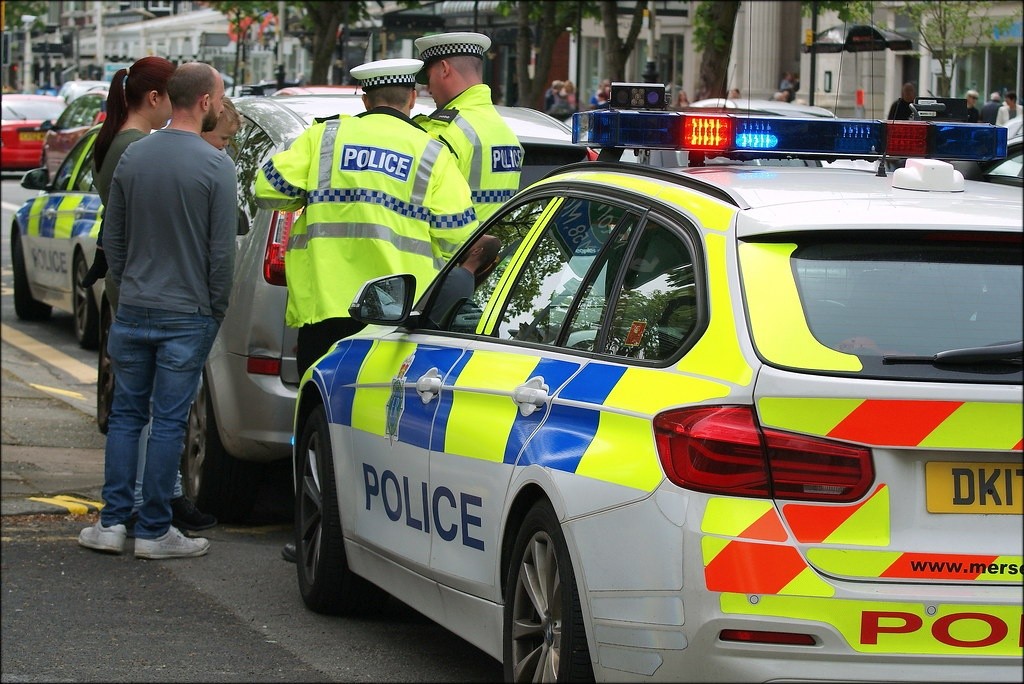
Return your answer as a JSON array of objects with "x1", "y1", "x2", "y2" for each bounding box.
[
  {"x1": 415, "y1": 31, "x2": 525, "y2": 258},
  {"x1": 91, "y1": 55, "x2": 217, "y2": 536},
  {"x1": 888, "y1": 83, "x2": 915, "y2": 120},
  {"x1": 981, "y1": 92, "x2": 1002, "y2": 125},
  {"x1": 545, "y1": 79, "x2": 576, "y2": 119},
  {"x1": 78, "y1": 62, "x2": 237, "y2": 558},
  {"x1": 81, "y1": 96, "x2": 241, "y2": 284},
  {"x1": 965, "y1": 90, "x2": 979, "y2": 122},
  {"x1": 257, "y1": 58, "x2": 479, "y2": 560},
  {"x1": 769, "y1": 71, "x2": 800, "y2": 102},
  {"x1": 996, "y1": 93, "x2": 1023, "y2": 126},
  {"x1": 429, "y1": 235, "x2": 503, "y2": 323},
  {"x1": 589, "y1": 79, "x2": 611, "y2": 109},
  {"x1": 731, "y1": 89, "x2": 741, "y2": 98},
  {"x1": 676, "y1": 90, "x2": 690, "y2": 107}
]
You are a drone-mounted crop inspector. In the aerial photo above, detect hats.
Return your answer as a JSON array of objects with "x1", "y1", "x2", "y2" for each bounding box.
[
  {"x1": 416, "y1": 32, "x2": 492, "y2": 85},
  {"x1": 350, "y1": 57, "x2": 424, "y2": 92}
]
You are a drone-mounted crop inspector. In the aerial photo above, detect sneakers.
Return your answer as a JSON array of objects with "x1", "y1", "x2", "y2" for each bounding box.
[
  {"x1": 171, "y1": 497, "x2": 216, "y2": 530},
  {"x1": 78, "y1": 518, "x2": 127, "y2": 554},
  {"x1": 135, "y1": 524, "x2": 210, "y2": 559}
]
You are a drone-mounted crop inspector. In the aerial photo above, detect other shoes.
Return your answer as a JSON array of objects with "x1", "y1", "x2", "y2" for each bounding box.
[{"x1": 281, "y1": 540, "x2": 297, "y2": 561}]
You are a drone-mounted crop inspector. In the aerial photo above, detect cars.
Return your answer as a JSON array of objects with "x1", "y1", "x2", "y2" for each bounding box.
[
  {"x1": 0, "y1": 92, "x2": 68, "y2": 171},
  {"x1": 289, "y1": 105, "x2": 1021, "y2": 682},
  {"x1": 10, "y1": 81, "x2": 839, "y2": 518}
]
